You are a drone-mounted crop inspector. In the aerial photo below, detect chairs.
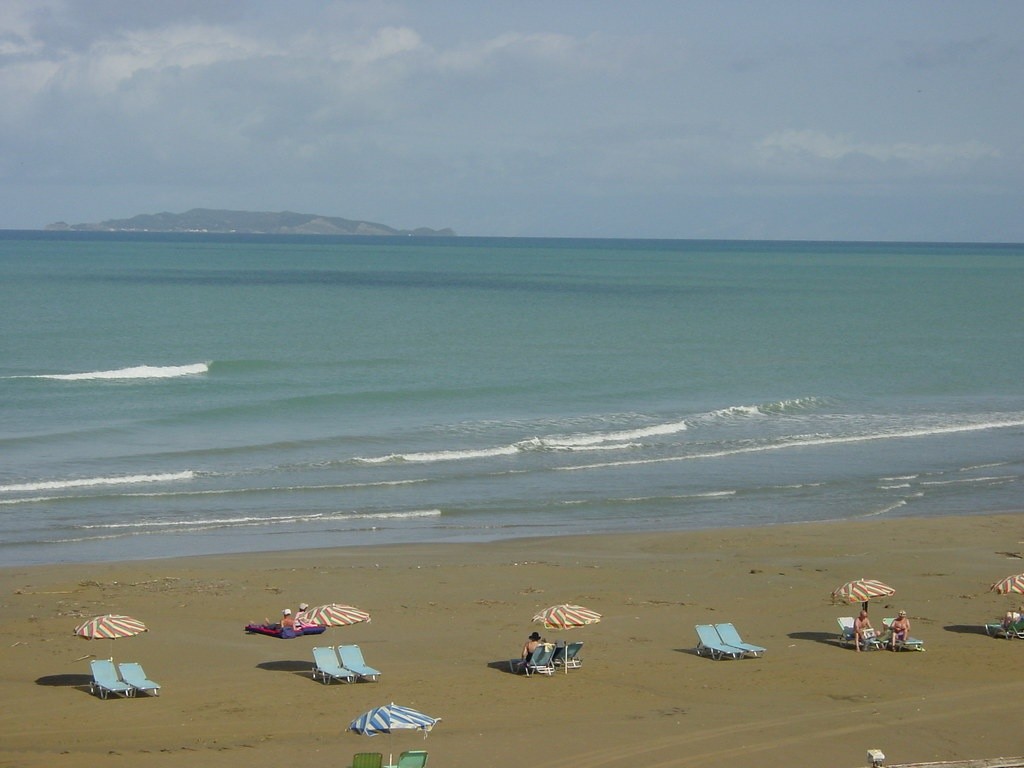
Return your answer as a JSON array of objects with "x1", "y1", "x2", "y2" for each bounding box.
[
  {"x1": 310, "y1": 644, "x2": 381, "y2": 684},
  {"x1": 508, "y1": 642, "x2": 585, "y2": 677},
  {"x1": 351, "y1": 751, "x2": 428, "y2": 768},
  {"x1": 985, "y1": 616, "x2": 1024, "y2": 641},
  {"x1": 695, "y1": 622, "x2": 768, "y2": 660},
  {"x1": 837, "y1": 616, "x2": 924, "y2": 654},
  {"x1": 90, "y1": 659, "x2": 159, "y2": 701}
]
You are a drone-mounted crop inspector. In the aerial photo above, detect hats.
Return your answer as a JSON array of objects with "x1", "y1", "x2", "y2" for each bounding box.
[
  {"x1": 529, "y1": 632, "x2": 540, "y2": 640},
  {"x1": 282, "y1": 609, "x2": 291, "y2": 615},
  {"x1": 299, "y1": 603, "x2": 308, "y2": 610}
]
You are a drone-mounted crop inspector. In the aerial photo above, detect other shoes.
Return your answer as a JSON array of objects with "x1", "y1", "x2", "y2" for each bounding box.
[{"x1": 892, "y1": 646, "x2": 897, "y2": 652}]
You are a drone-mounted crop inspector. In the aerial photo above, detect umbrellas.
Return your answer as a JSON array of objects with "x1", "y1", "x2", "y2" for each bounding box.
[
  {"x1": 988, "y1": 573, "x2": 1024, "y2": 596},
  {"x1": 830, "y1": 577, "x2": 896, "y2": 612},
  {"x1": 531, "y1": 603, "x2": 603, "y2": 675},
  {"x1": 73, "y1": 613, "x2": 150, "y2": 663},
  {"x1": 298, "y1": 603, "x2": 372, "y2": 628},
  {"x1": 344, "y1": 701, "x2": 442, "y2": 768}
]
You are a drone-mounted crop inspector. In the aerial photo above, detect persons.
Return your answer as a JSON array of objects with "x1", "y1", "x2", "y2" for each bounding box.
[
  {"x1": 854, "y1": 611, "x2": 881, "y2": 652},
  {"x1": 522, "y1": 632, "x2": 546, "y2": 663},
  {"x1": 265, "y1": 602, "x2": 310, "y2": 626},
  {"x1": 890, "y1": 611, "x2": 911, "y2": 652},
  {"x1": 1000, "y1": 617, "x2": 1019, "y2": 627},
  {"x1": 250, "y1": 608, "x2": 297, "y2": 631}
]
